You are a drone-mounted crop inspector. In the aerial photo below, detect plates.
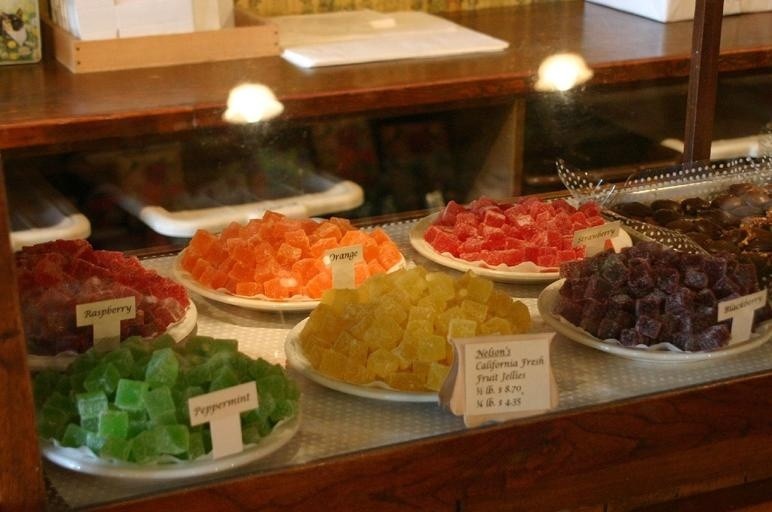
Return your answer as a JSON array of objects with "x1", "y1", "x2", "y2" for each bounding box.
[
  {"x1": 284, "y1": 316, "x2": 440, "y2": 404},
  {"x1": 173, "y1": 243, "x2": 402, "y2": 314},
  {"x1": 19, "y1": 272, "x2": 197, "y2": 369},
  {"x1": 537, "y1": 276, "x2": 767, "y2": 365},
  {"x1": 407, "y1": 207, "x2": 632, "y2": 284},
  {"x1": 35, "y1": 407, "x2": 302, "y2": 480}
]
